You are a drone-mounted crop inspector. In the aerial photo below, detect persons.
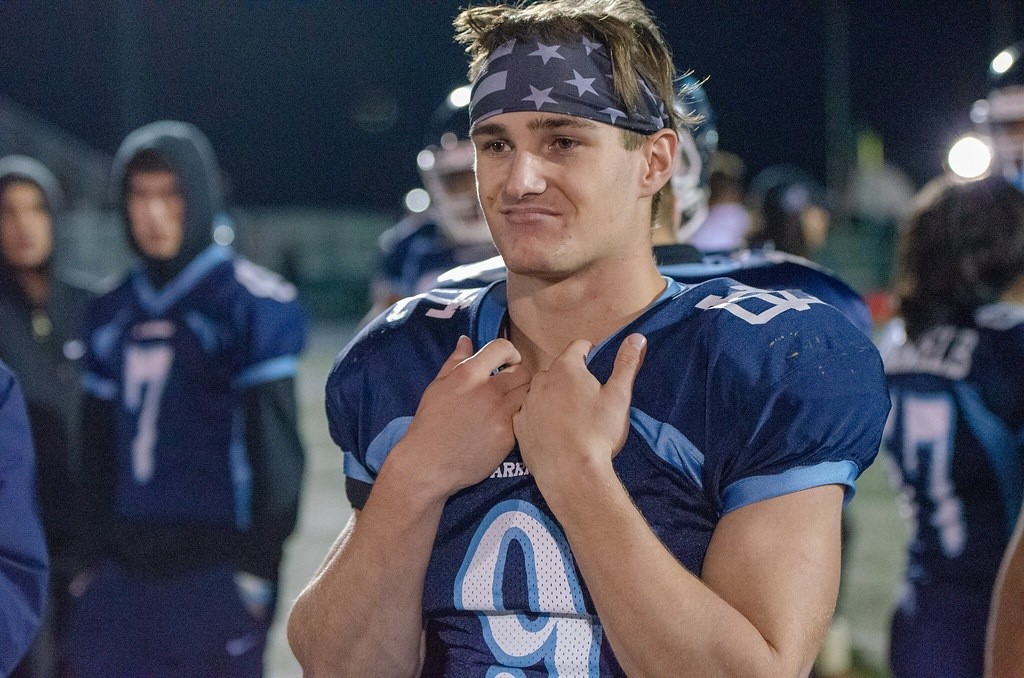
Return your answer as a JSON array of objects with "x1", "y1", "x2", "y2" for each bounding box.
[{"x1": 0, "y1": 0, "x2": 1024, "y2": 678}]
